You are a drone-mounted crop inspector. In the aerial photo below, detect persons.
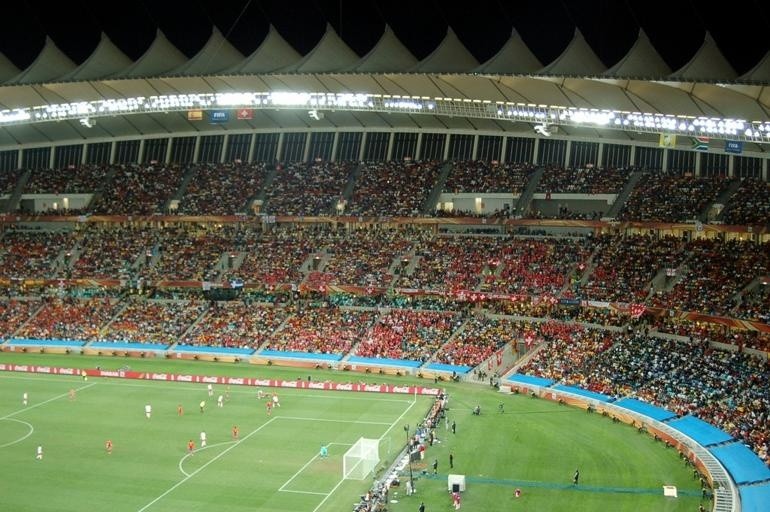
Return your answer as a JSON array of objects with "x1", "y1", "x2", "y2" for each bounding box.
[{"x1": 0, "y1": 157, "x2": 770, "y2": 511}]
